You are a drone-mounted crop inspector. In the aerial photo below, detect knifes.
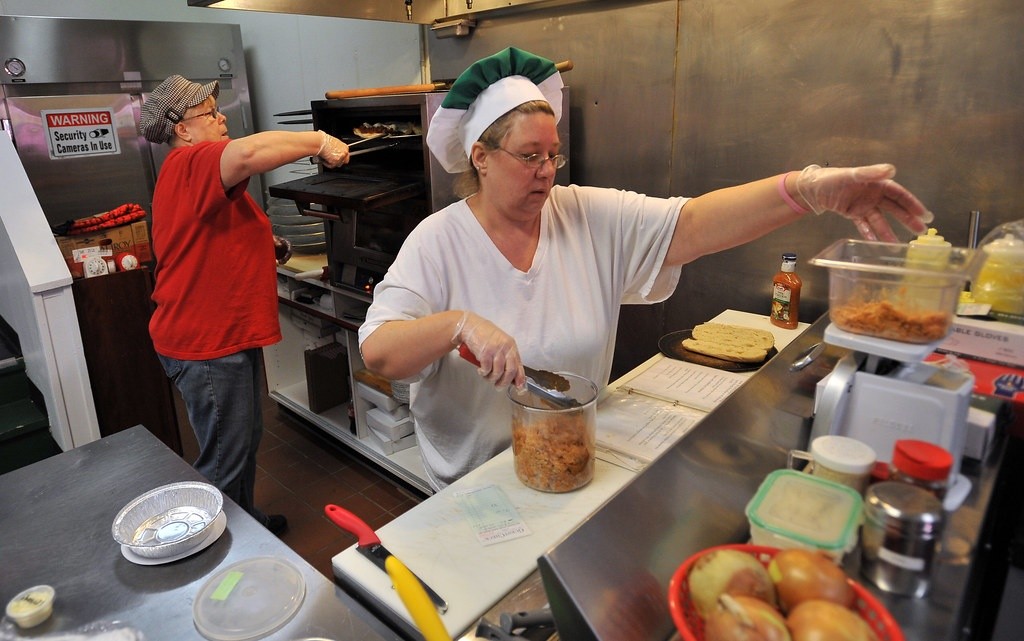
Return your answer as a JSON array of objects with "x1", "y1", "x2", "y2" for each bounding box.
[{"x1": 325, "y1": 504, "x2": 448, "y2": 614}]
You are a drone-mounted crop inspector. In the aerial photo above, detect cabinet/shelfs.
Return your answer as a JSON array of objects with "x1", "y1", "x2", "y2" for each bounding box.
[{"x1": 260, "y1": 254, "x2": 436, "y2": 495}]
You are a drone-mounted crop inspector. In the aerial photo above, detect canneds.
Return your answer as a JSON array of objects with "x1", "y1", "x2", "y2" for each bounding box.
[{"x1": 891, "y1": 440, "x2": 953, "y2": 503}]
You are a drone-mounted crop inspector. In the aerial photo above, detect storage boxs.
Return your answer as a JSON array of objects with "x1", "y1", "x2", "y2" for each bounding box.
[
  {"x1": 745, "y1": 468, "x2": 863, "y2": 566},
  {"x1": 57, "y1": 220, "x2": 152, "y2": 277},
  {"x1": 920, "y1": 310, "x2": 1024, "y2": 403},
  {"x1": 809, "y1": 237, "x2": 989, "y2": 343}
]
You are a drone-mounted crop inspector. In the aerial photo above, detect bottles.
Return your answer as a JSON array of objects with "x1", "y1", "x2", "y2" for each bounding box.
[
  {"x1": 889, "y1": 438, "x2": 954, "y2": 505},
  {"x1": 899, "y1": 229, "x2": 951, "y2": 306},
  {"x1": 861, "y1": 482, "x2": 942, "y2": 596},
  {"x1": 770, "y1": 253, "x2": 802, "y2": 328}
]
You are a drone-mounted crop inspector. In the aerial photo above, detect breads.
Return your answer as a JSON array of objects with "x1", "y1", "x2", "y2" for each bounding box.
[
  {"x1": 352, "y1": 120, "x2": 422, "y2": 138},
  {"x1": 285, "y1": 255, "x2": 329, "y2": 272},
  {"x1": 681, "y1": 322, "x2": 775, "y2": 363}
]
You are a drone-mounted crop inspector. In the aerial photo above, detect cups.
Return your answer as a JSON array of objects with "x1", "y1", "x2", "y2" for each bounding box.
[
  {"x1": 789, "y1": 435, "x2": 873, "y2": 497},
  {"x1": 508, "y1": 372, "x2": 599, "y2": 492}
]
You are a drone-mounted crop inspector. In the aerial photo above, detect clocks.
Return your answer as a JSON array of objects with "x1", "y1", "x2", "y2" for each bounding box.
[
  {"x1": 115, "y1": 252, "x2": 141, "y2": 272},
  {"x1": 84, "y1": 252, "x2": 109, "y2": 278}
]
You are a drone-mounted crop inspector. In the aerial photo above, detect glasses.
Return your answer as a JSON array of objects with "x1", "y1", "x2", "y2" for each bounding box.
[
  {"x1": 180, "y1": 106, "x2": 222, "y2": 122},
  {"x1": 477, "y1": 140, "x2": 568, "y2": 171}
]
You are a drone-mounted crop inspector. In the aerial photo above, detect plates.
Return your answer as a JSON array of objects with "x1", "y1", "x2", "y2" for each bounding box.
[
  {"x1": 658, "y1": 328, "x2": 777, "y2": 372},
  {"x1": 273, "y1": 201, "x2": 327, "y2": 255}
]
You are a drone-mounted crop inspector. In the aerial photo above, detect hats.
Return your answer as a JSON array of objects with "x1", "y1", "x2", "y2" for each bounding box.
[
  {"x1": 140, "y1": 75, "x2": 220, "y2": 145},
  {"x1": 426, "y1": 45, "x2": 564, "y2": 174}
]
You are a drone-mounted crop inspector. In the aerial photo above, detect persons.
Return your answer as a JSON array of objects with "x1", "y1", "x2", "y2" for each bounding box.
[
  {"x1": 359, "y1": 45, "x2": 934, "y2": 494},
  {"x1": 139, "y1": 74, "x2": 349, "y2": 536}
]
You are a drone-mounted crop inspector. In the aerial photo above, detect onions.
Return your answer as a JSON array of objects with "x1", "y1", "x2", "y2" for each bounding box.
[{"x1": 688, "y1": 549, "x2": 878, "y2": 641}]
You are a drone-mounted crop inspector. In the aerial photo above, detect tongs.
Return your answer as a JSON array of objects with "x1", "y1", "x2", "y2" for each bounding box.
[
  {"x1": 456, "y1": 344, "x2": 586, "y2": 418},
  {"x1": 309, "y1": 132, "x2": 402, "y2": 164}
]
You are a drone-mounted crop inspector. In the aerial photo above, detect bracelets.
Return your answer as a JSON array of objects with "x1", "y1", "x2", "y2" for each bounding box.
[{"x1": 778, "y1": 171, "x2": 810, "y2": 214}]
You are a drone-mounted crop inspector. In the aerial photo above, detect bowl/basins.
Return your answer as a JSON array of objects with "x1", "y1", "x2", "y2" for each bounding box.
[
  {"x1": 4, "y1": 583, "x2": 56, "y2": 629},
  {"x1": 808, "y1": 237, "x2": 988, "y2": 343},
  {"x1": 110, "y1": 480, "x2": 223, "y2": 557}
]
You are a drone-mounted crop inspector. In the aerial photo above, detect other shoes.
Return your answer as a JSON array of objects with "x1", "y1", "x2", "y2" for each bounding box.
[{"x1": 255, "y1": 513, "x2": 288, "y2": 536}]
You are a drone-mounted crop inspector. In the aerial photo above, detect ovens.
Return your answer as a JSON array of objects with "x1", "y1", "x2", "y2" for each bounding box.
[{"x1": 268, "y1": 85, "x2": 570, "y2": 301}]
